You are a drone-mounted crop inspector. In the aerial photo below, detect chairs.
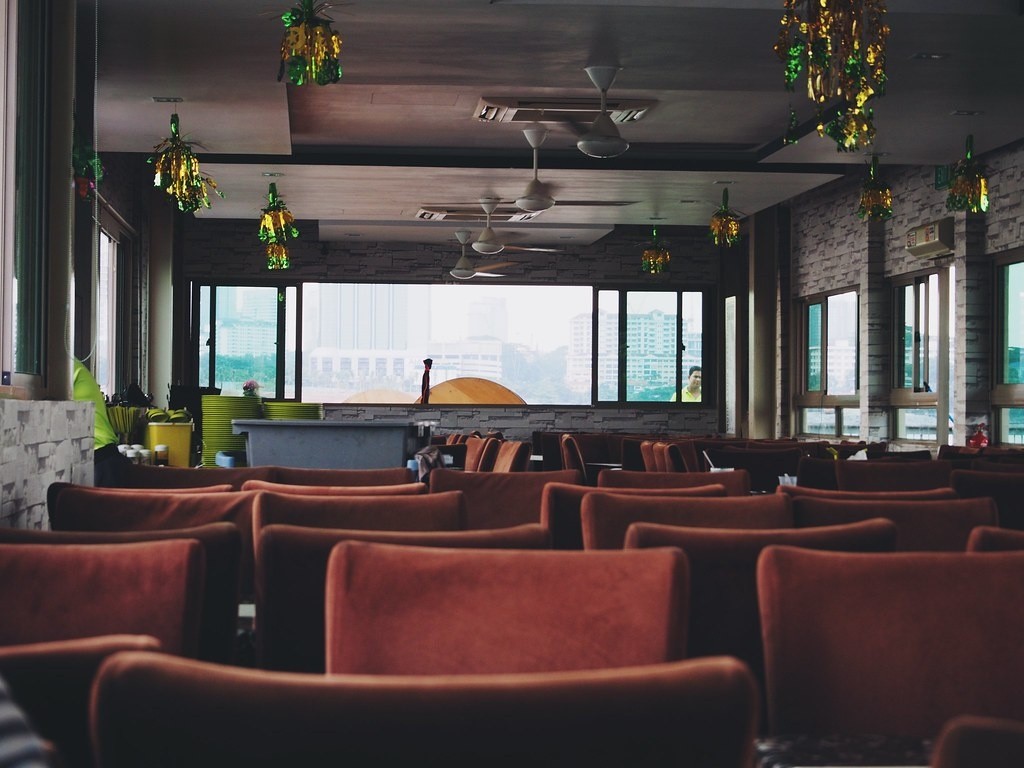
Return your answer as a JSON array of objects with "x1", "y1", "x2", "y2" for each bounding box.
[{"x1": 0, "y1": 429, "x2": 1024, "y2": 768}]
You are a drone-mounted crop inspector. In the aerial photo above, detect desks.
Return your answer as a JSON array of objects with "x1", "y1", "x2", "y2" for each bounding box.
[{"x1": 231, "y1": 419, "x2": 414, "y2": 469}]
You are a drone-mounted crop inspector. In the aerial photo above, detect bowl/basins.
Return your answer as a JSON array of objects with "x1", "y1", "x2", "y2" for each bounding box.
[{"x1": 145, "y1": 409, "x2": 192, "y2": 423}]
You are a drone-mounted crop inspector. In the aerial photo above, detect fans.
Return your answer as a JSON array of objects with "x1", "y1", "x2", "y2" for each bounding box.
[
  {"x1": 421, "y1": 124, "x2": 639, "y2": 211},
  {"x1": 559, "y1": 65, "x2": 629, "y2": 159},
  {"x1": 380, "y1": 197, "x2": 565, "y2": 254},
  {"x1": 375, "y1": 231, "x2": 506, "y2": 280}
]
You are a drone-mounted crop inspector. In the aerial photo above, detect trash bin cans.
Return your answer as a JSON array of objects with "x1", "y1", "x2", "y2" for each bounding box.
[{"x1": 215, "y1": 450, "x2": 247, "y2": 468}]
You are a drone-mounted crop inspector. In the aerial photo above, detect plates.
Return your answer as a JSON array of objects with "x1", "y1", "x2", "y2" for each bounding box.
[{"x1": 201, "y1": 395, "x2": 324, "y2": 469}]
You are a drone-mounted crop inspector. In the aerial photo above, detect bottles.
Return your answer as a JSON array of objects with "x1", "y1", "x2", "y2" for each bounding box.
[
  {"x1": 154, "y1": 444, "x2": 169, "y2": 466},
  {"x1": 968, "y1": 422, "x2": 989, "y2": 448},
  {"x1": 118, "y1": 443, "x2": 152, "y2": 467}
]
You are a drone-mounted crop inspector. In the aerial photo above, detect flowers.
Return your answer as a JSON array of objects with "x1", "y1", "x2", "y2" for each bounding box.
[{"x1": 243, "y1": 379, "x2": 260, "y2": 396}]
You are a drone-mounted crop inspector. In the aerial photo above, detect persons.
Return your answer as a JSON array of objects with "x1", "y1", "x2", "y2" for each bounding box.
[
  {"x1": 72, "y1": 356, "x2": 125, "y2": 489},
  {"x1": 671, "y1": 366, "x2": 702, "y2": 402}
]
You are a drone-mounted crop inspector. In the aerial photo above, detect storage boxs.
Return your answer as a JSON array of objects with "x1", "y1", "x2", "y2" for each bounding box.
[{"x1": 143, "y1": 422, "x2": 192, "y2": 467}]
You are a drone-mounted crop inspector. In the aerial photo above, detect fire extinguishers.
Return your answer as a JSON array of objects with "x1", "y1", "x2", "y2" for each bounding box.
[{"x1": 969, "y1": 423, "x2": 989, "y2": 448}]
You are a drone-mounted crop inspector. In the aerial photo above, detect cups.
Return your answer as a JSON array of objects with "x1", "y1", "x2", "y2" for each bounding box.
[{"x1": 778, "y1": 476, "x2": 797, "y2": 487}]
